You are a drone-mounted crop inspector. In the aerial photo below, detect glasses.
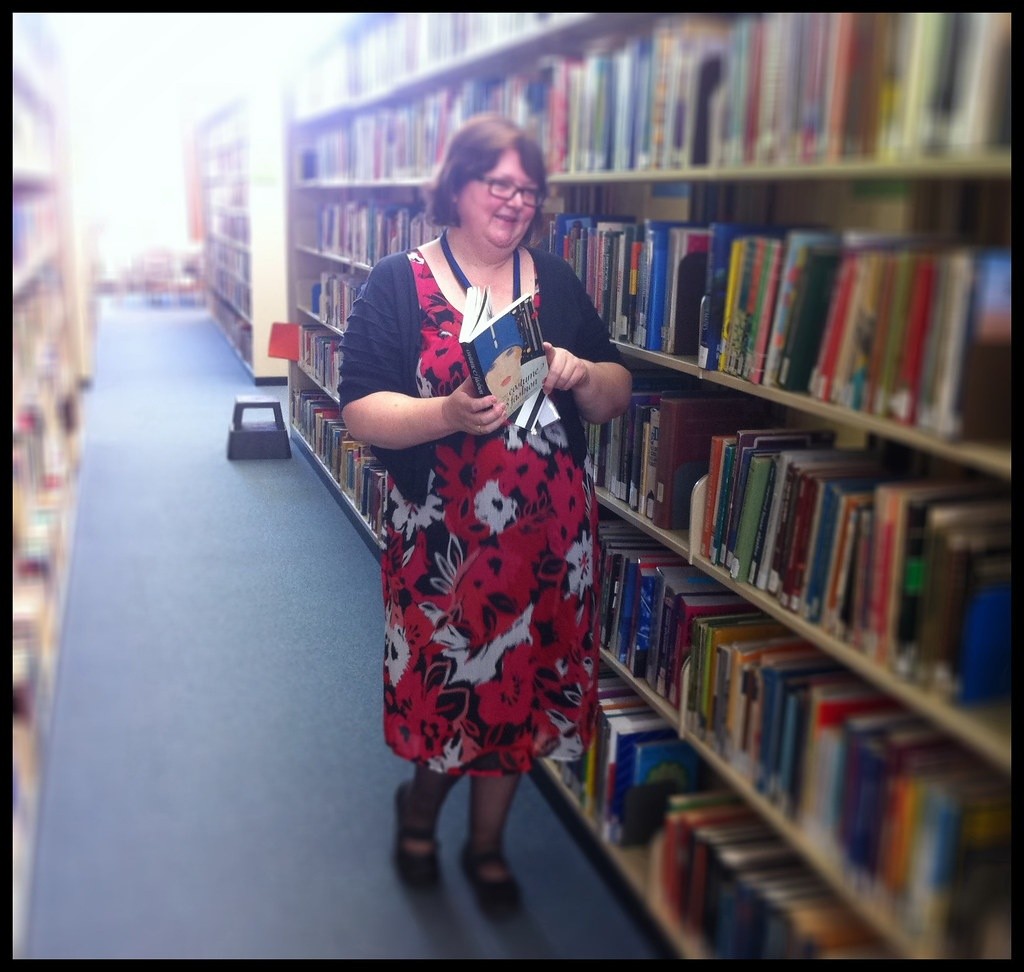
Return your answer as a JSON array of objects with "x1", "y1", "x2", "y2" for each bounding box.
[{"x1": 471, "y1": 172, "x2": 548, "y2": 207}]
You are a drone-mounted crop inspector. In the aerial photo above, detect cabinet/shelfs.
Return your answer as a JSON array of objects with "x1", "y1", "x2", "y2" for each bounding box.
[
  {"x1": 13, "y1": 60, "x2": 92, "y2": 959},
  {"x1": 203, "y1": 101, "x2": 287, "y2": 386},
  {"x1": 287, "y1": 15, "x2": 1011, "y2": 959}
]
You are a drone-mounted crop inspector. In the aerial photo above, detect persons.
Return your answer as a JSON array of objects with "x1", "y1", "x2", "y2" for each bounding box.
[{"x1": 333, "y1": 111, "x2": 635, "y2": 923}]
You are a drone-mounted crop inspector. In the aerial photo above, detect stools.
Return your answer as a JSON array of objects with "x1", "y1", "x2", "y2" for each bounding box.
[{"x1": 227, "y1": 395, "x2": 292, "y2": 459}]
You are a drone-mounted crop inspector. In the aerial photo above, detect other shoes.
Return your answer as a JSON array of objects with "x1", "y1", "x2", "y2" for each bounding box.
[
  {"x1": 460, "y1": 847, "x2": 519, "y2": 909},
  {"x1": 394, "y1": 783, "x2": 438, "y2": 892}
]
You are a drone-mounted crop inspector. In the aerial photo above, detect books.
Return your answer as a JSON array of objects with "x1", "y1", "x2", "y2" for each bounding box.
[
  {"x1": 12, "y1": 94, "x2": 82, "y2": 833},
  {"x1": 526, "y1": 14, "x2": 1011, "y2": 961},
  {"x1": 197, "y1": 144, "x2": 251, "y2": 365},
  {"x1": 459, "y1": 284, "x2": 549, "y2": 422},
  {"x1": 287, "y1": 11, "x2": 520, "y2": 542}
]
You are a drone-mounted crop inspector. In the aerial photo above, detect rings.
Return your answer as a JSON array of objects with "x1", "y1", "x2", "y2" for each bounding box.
[{"x1": 477, "y1": 425, "x2": 483, "y2": 434}]
